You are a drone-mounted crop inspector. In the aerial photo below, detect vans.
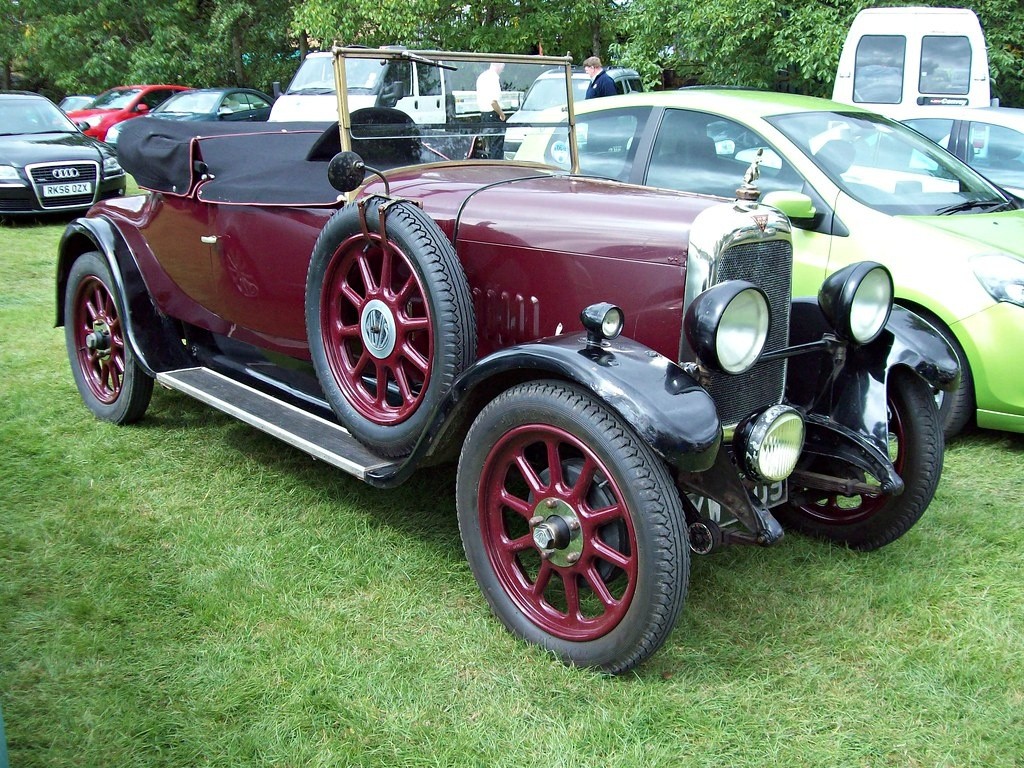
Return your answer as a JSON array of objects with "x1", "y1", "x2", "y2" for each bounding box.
[
  {"x1": 832, "y1": 8, "x2": 998, "y2": 171},
  {"x1": 267, "y1": 43, "x2": 560, "y2": 159}
]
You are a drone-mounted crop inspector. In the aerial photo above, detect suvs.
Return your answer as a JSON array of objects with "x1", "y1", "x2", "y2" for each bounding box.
[{"x1": 505, "y1": 67, "x2": 644, "y2": 160}]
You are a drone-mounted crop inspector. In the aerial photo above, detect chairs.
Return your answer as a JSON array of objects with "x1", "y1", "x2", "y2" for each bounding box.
[
  {"x1": 674, "y1": 134, "x2": 722, "y2": 168},
  {"x1": 228, "y1": 100, "x2": 240, "y2": 112},
  {"x1": 984, "y1": 136, "x2": 1024, "y2": 170},
  {"x1": 815, "y1": 142, "x2": 861, "y2": 175}
]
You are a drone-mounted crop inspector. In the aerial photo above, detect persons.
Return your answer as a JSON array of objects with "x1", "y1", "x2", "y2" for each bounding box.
[
  {"x1": 583, "y1": 56, "x2": 619, "y2": 100},
  {"x1": 475, "y1": 63, "x2": 506, "y2": 161}
]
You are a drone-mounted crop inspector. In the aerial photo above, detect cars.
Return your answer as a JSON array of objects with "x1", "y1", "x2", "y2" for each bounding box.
[
  {"x1": 514, "y1": 87, "x2": 1024, "y2": 441},
  {"x1": 105, "y1": 88, "x2": 276, "y2": 148},
  {"x1": 735, "y1": 106, "x2": 1024, "y2": 199},
  {"x1": 53, "y1": 50, "x2": 959, "y2": 676},
  {"x1": 0, "y1": 92, "x2": 127, "y2": 219},
  {"x1": 53, "y1": 84, "x2": 199, "y2": 143}
]
